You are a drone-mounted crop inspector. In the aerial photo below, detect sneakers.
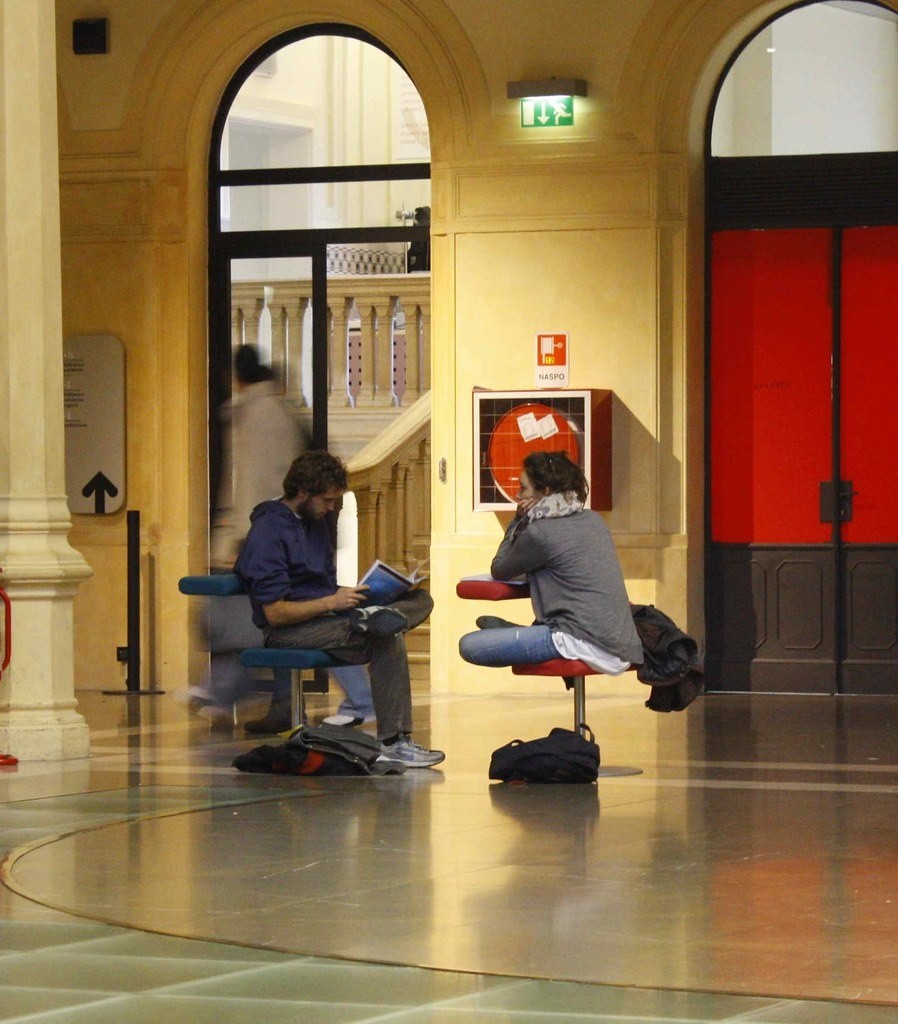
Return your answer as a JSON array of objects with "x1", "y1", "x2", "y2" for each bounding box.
[
  {"x1": 376, "y1": 732, "x2": 445, "y2": 767},
  {"x1": 350, "y1": 605, "x2": 408, "y2": 640},
  {"x1": 322, "y1": 715, "x2": 377, "y2": 727}
]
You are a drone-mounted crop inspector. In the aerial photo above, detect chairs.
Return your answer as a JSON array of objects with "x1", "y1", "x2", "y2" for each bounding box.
[
  {"x1": 176, "y1": 574, "x2": 346, "y2": 726},
  {"x1": 456, "y1": 581, "x2": 644, "y2": 778}
]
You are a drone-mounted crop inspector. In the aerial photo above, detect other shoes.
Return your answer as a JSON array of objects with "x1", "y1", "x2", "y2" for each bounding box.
[
  {"x1": 476, "y1": 615, "x2": 528, "y2": 632},
  {"x1": 244, "y1": 716, "x2": 308, "y2": 734}
]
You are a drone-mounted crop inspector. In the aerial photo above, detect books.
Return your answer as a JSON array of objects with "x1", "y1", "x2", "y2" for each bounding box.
[{"x1": 356, "y1": 558, "x2": 429, "y2": 607}]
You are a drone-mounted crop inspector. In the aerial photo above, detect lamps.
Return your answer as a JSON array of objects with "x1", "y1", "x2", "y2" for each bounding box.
[{"x1": 506, "y1": 78, "x2": 587, "y2": 98}]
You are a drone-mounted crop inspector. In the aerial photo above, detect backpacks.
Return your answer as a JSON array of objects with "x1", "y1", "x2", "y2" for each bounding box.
[{"x1": 488, "y1": 722, "x2": 601, "y2": 784}]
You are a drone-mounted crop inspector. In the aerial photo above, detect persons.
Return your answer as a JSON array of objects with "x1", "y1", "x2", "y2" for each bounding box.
[
  {"x1": 458, "y1": 450, "x2": 645, "y2": 676},
  {"x1": 233, "y1": 451, "x2": 445, "y2": 769},
  {"x1": 183, "y1": 345, "x2": 309, "y2": 709}
]
